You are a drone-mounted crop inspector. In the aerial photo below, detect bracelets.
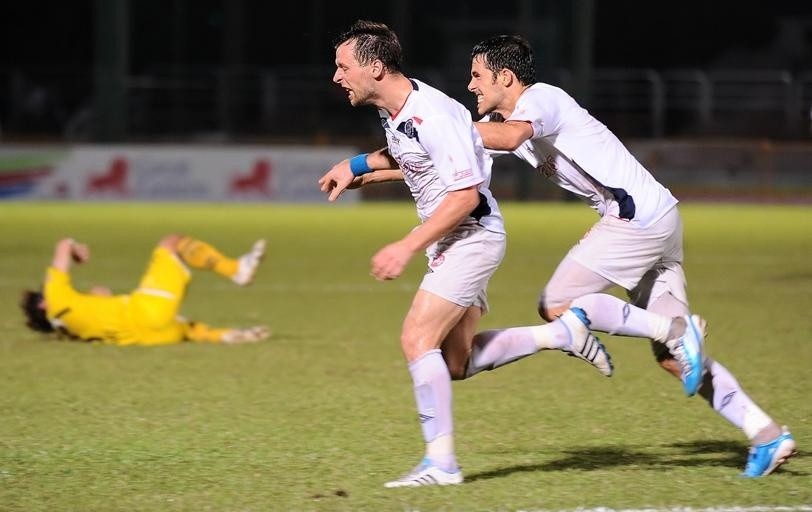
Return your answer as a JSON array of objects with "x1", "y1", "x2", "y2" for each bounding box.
[{"x1": 347, "y1": 154, "x2": 375, "y2": 176}]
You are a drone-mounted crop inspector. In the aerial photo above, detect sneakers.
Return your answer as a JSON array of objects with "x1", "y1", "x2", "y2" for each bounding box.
[
  {"x1": 245, "y1": 325, "x2": 270, "y2": 343},
  {"x1": 230, "y1": 239, "x2": 267, "y2": 286},
  {"x1": 665, "y1": 314, "x2": 709, "y2": 398},
  {"x1": 384, "y1": 455, "x2": 464, "y2": 489},
  {"x1": 558, "y1": 307, "x2": 614, "y2": 378},
  {"x1": 739, "y1": 425, "x2": 797, "y2": 477}
]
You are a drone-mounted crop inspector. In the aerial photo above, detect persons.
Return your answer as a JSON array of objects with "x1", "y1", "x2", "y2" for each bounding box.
[
  {"x1": 16, "y1": 231, "x2": 274, "y2": 351},
  {"x1": 317, "y1": 33, "x2": 798, "y2": 479},
  {"x1": 317, "y1": 20, "x2": 615, "y2": 489}
]
